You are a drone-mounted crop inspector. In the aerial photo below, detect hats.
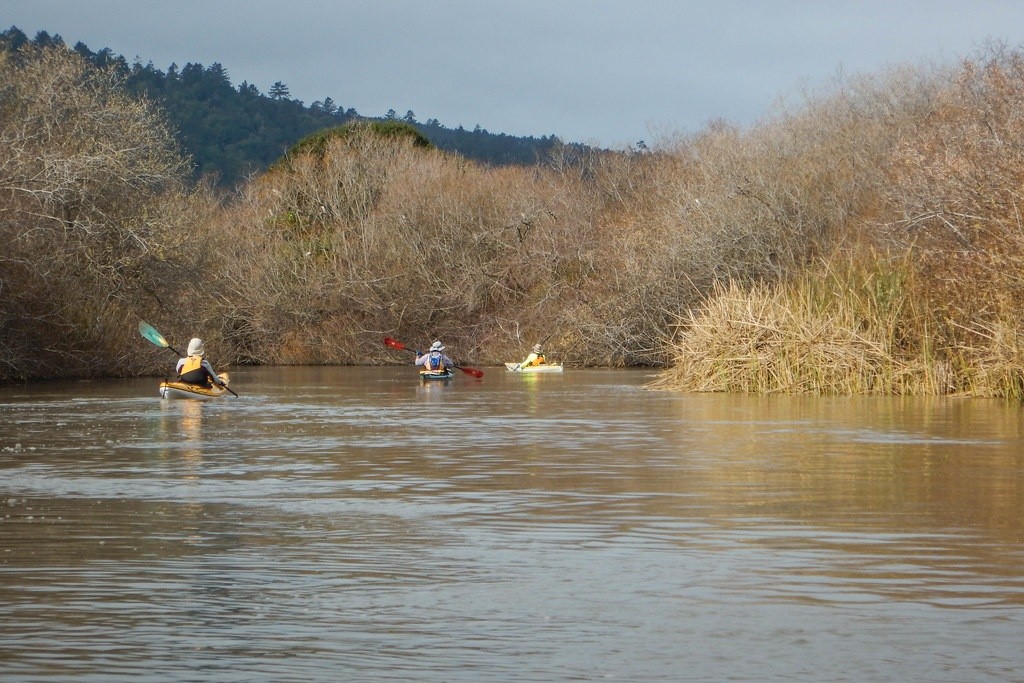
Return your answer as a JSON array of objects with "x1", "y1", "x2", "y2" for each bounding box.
[
  {"x1": 430, "y1": 341, "x2": 446, "y2": 351},
  {"x1": 532, "y1": 343, "x2": 542, "y2": 352},
  {"x1": 187, "y1": 338, "x2": 205, "y2": 357}
]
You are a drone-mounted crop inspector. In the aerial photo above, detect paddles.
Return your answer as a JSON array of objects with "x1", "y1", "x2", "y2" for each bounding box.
[
  {"x1": 138, "y1": 321, "x2": 239, "y2": 398},
  {"x1": 514, "y1": 334, "x2": 551, "y2": 372},
  {"x1": 383, "y1": 337, "x2": 485, "y2": 379}
]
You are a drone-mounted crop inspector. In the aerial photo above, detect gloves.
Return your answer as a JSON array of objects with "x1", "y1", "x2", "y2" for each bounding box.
[
  {"x1": 416, "y1": 351, "x2": 422, "y2": 356},
  {"x1": 220, "y1": 382, "x2": 225, "y2": 386}
]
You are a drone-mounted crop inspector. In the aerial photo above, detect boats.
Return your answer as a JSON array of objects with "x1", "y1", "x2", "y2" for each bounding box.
[
  {"x1": 419, "y1": 368, "x2": 456, "y2": 381},
  {"x1": 503, "y1": 360, "x2": 565, "y2": 373},
  {"x1": 158, "y1": 370, "x2": 230, "y2": 399}
]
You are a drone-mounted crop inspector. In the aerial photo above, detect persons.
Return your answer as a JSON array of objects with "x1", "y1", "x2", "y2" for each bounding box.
[
  {"x1": 415, "y1": 341, "x2": 454, "y2": 370},
  {"x1": 520, "y1": 344, "x2": 546, "y2": 368},
  {"x1": 176, "y1": 338, "x2": 221, "y2": 387}
]
